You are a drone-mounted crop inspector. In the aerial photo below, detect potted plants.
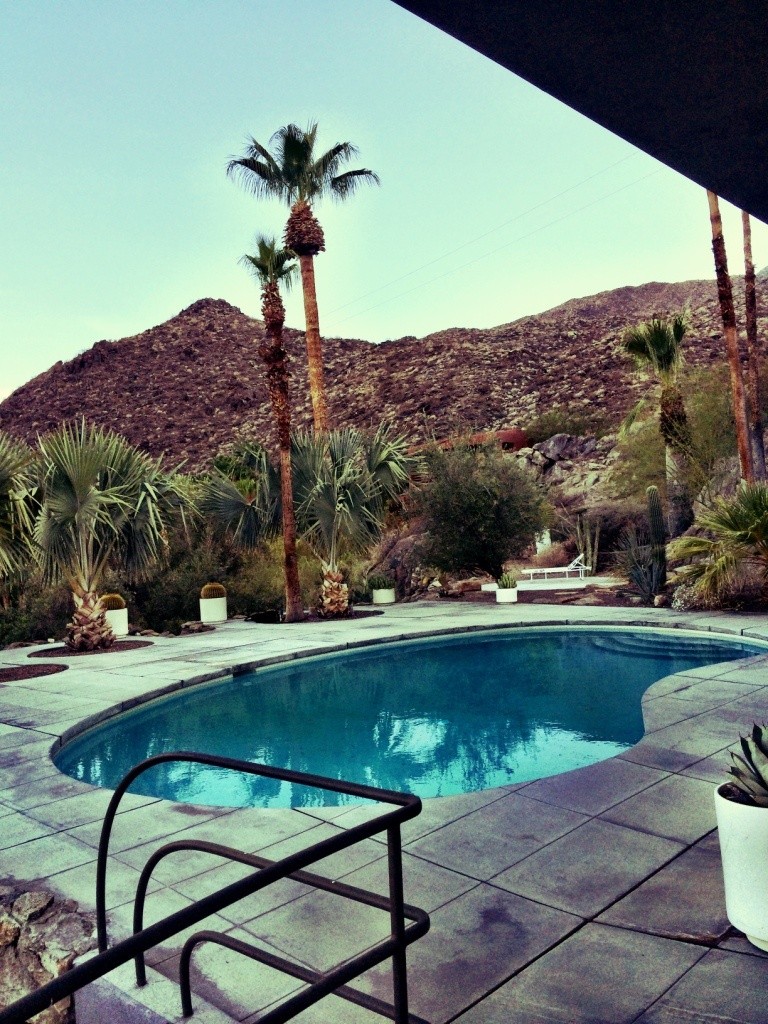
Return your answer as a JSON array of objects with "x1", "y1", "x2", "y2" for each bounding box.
[
  {"x1": 496, "y1": 572, "x2": 518, "y2": 603},
  {"x1": 714, "y1": 719, "x2": 768, "y2": 951},
  {"x1": 101, "y1": 593, "x2": 129, "y2": 637},
  {"x1": 369, "y1": 574, "x2": 396, "y2": 603},
  {"x1": 199, "y1": 582, "x2": 227, "y2": 624}
]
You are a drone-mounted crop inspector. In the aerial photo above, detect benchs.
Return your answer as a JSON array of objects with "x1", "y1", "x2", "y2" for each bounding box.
[{"x1": 520, "y1": 553, "x2": 592, "y2": 580}]
